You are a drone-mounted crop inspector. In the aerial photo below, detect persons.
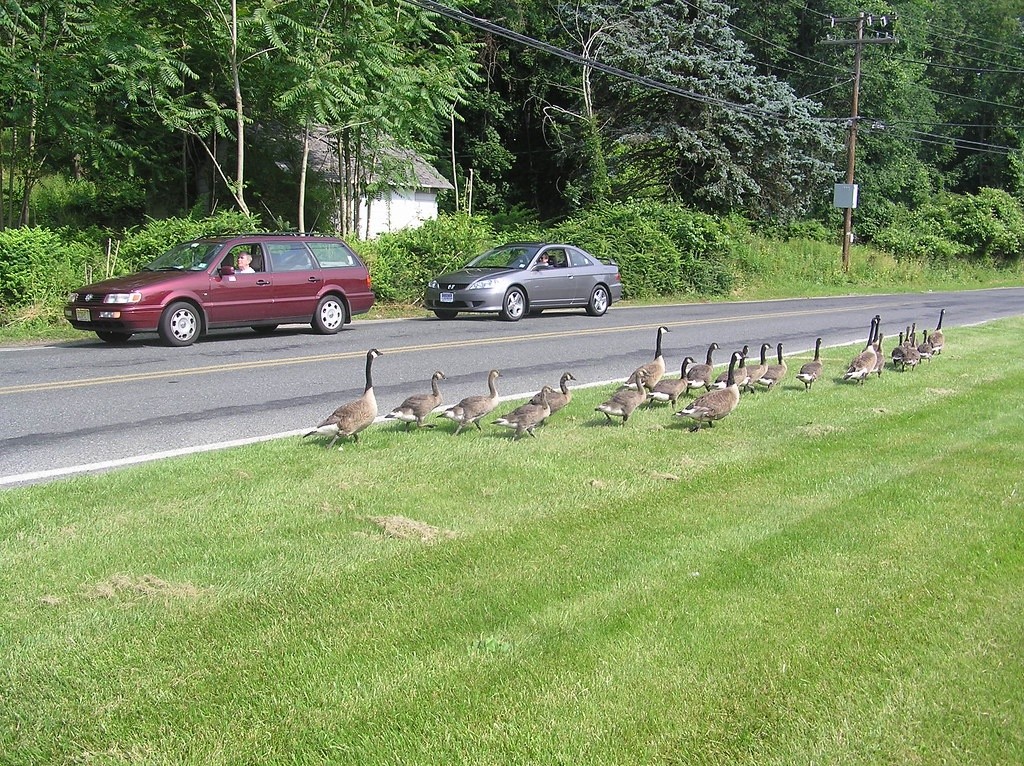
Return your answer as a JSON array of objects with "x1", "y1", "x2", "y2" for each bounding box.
[
  {"x1": 235, "y1": 251, "x2": 255, "y2": 273},
  {"x1": 538, "y1": 252, "x2": 553, "y2": 267}
]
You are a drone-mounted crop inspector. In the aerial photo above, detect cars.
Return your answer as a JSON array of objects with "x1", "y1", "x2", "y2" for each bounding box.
[{"x1": 423, "y1": 241, "x2": 622, "y2": 322}]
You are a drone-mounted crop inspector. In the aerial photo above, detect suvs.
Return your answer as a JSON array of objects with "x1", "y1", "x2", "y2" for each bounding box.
[{"x1": 63, "y1": 233, "x2": 375, "y2": 348}]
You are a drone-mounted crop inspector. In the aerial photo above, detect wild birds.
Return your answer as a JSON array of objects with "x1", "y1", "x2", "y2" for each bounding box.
[
  {"x1": 595, "y1": 368, "x2": 651, "y2": 426},
  {"x1": 436, "y1": 369, "x2": 503, "y2": 437},
  {"x1": 643, "y1": 357, "x2": 697, "y2": 413},
  {"x1": 712, "y1": 343, "x2": 773, "y2": 394},
  {"x1": 673, "y1": 351, "x2": 749, "y2": 432},
  {"x1": 795, "y1": 337, "x2": 823, "y2": 389},
  {"x1": 384, "y1": 370, "x2": 448, "y2": 434},
  {"x1": 685, "y1": 343, "x2": 721, "y2": 396},
  {"x1": 529, "y1": 372, "x2": 577, "y2": 426},
  {"x1": 842, "y1": 315, "x2": 885, "y2": 386},
  {"x1": 891, "y1": 323, "x2": 920, "y2": 373},
  {"x1": 928, "y1": 308, "x2": 945, "y2": 355},
  {"x1": 303, "y1": 348, "x2": 383, "y2": 449},
  {"x1": 759, "y1": 342, "x2": 787, "y2": 393},
  {"x1": 917, "y1": 330, "x2": 934, "y2": 363},
  {"x1": 625, "y1": 326, "x2": 671, "y2": 400},
  {"x1": 490, "y1": 385, "x2": 554, "y2": 443}
]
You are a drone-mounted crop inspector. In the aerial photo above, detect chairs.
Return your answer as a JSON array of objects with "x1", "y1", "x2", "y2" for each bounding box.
[
  {"x1": 220, "y1": 253, "x2": 234, "y2": 269},
  {"x1": 547, "y1": 255, "x2": 558, "y2": 268},
  {"x1": 249, "y1": 253, "x2": 262, "y2": 273}
]
